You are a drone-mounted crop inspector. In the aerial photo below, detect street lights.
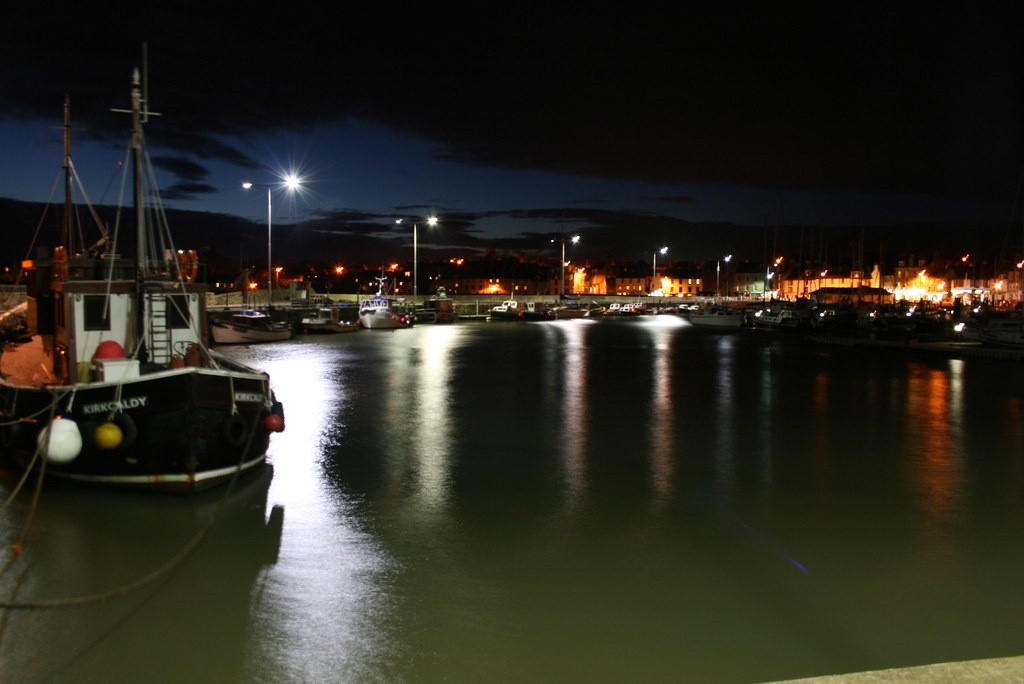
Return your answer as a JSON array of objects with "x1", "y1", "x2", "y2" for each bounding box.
[
  {"x1": 550, "y1": 234, "x2": 580, "y2": 293},
  {"x1": 240, "y1": 170, "x2": 306, "y2": 307},
  {"x1": 396, "y1": 216, "x2": 439, "y2": 302},
  {"x1": 652, "y1": 243, "x2": 668, "y2": 293}
]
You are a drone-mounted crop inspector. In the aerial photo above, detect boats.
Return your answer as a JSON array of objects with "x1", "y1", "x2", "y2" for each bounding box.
[
  {"x1": 208, "y1": 308, "x2": 272, "y2": 324},
  {"x1": 358, "y1": 276, "x2": 411, "y2": 329},
  {"x1": 972, "y1": 305, "x2": 1024, "y2": 349},
  {"x1": 416, "y1": 286, "x2": 456, "y2": 323},
  {"x1": 490, "y1": 283, "x2": 523, "y2": 318},
  {"x1": 523, "y1": 302, "x2": 547, "y2": 319},
  {"x1": 0, "y1": 38, "x2": 287, "y2": 499},
  {"x1": 689, "y1": 306, "x2": 746, "y2": 327},
  {"x1": 209, "y1": 315, "x2": 293, "y2": 346},
  {"x1": 301, "y1": 308, "x2": 358, "y2": 333},
  {"x1": 607, "y1": 303, "x2": 648, "y2": 315},
  {"x1": 752, "y1": 302, "x2": 801, "y2": 329}
]
